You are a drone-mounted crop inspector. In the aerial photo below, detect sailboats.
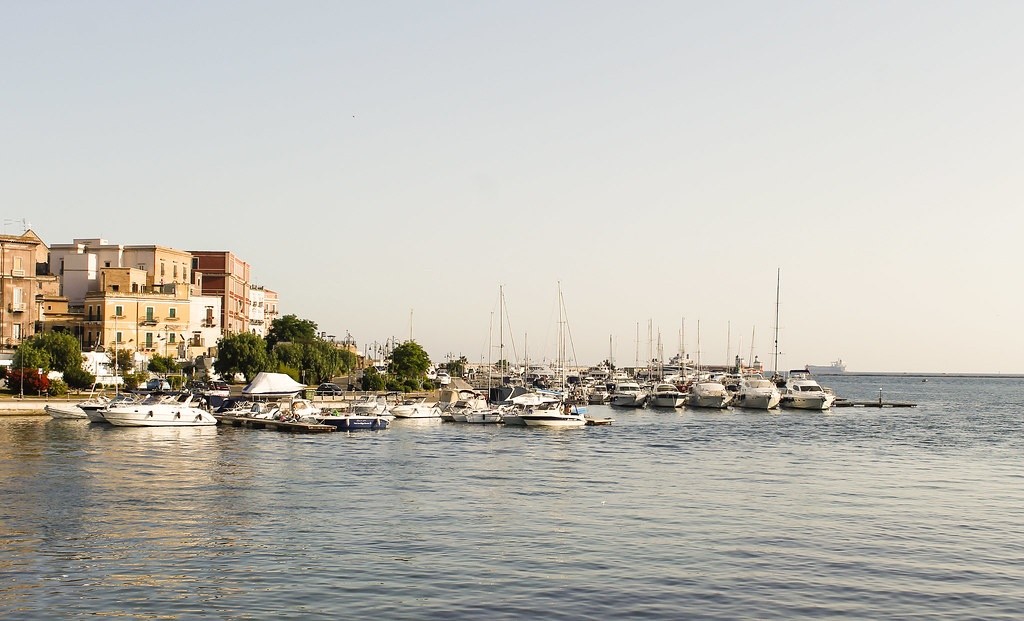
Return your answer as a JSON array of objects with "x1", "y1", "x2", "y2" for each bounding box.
[{"x1": 439, "y1": 267, "x2": 828, "y2": 427}]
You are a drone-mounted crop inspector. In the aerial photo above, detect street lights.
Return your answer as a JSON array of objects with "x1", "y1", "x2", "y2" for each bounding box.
[
  {"x1": 344, "y1": 329, "x2": 355, "y2": 388},
  {"x1": 368, "y1": 334, "x2": 401, "y2": 370}
]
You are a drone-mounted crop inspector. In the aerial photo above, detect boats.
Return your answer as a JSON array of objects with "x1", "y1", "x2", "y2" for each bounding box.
[
  {"x1": 40, "y1": 389, "x2": 442, "y2": 433},
  {"x1": 803, "y1": 357, "x2": 847, "y2": 376}
]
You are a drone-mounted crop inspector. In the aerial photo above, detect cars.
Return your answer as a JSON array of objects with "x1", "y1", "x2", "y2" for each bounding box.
[
  {"x1": 317, "y1": 382, "x2": 343, "y2": 396},
  {"x1": 434, "y1": 371, "x2": 452, "y2": 385},
  {"x1": 146, "y1": 378, "x2": 171, "y2": 391},
  {"x1": 203, "y1": 381, "x2": 232, "y2": 401}
]
